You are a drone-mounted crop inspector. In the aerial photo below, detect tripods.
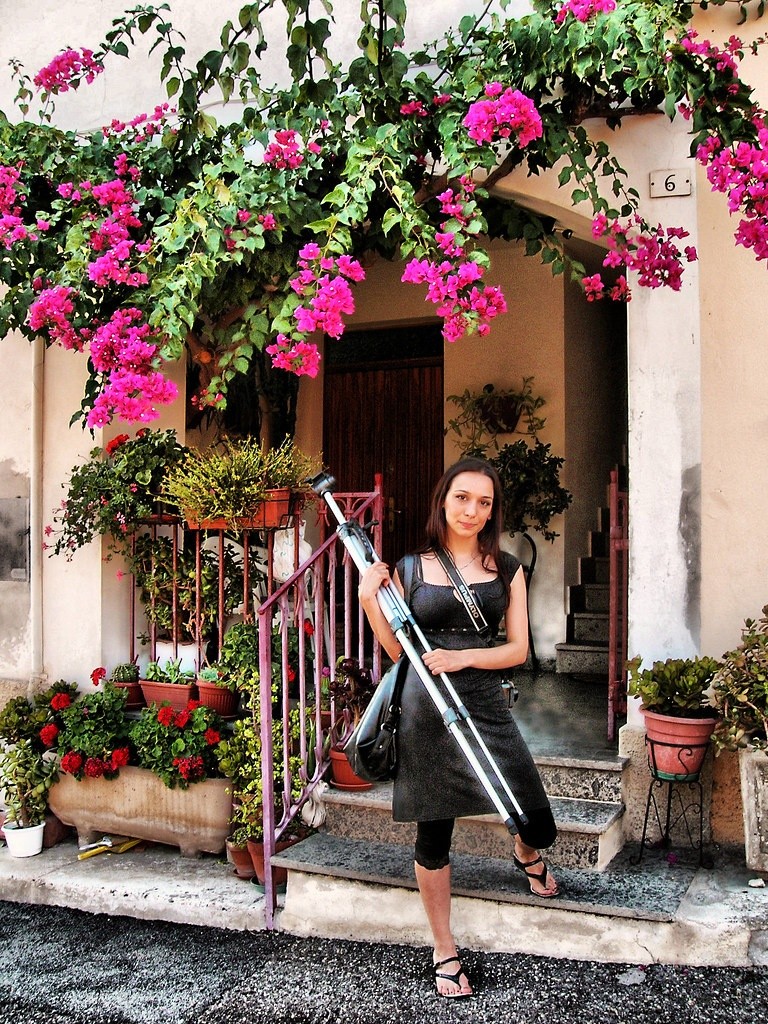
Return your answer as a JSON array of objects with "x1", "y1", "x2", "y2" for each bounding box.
[{"x1": 308, "y1": 470, "x2": 528, "y2": 836}]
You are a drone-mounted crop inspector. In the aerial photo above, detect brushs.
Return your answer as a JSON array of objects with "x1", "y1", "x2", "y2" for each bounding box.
[{"x1": 78, "y1": 834, "x2": 130, "y2": 851}]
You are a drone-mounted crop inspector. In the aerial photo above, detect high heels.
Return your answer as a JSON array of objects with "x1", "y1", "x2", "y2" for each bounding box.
[
  {"x1": 514, "y1": 852, "x2": 559, "y2": 897},
  {"x1": 431, "y1": 950, "x2": 473, "y2": 999}
]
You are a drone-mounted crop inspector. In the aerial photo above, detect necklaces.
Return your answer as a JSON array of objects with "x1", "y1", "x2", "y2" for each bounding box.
[{"x1": 460, "y1": 556, "x2": 477, "y2": 570}]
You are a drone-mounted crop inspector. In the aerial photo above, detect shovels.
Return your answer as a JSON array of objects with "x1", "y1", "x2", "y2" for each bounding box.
[{"x1": 77, "y1": 838, "x2": 142, "y2": 860}]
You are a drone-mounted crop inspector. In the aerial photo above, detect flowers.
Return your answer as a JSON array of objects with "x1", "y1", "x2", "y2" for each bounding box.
[
  {"x1": 0, "y1": 0, "x2": 768, "y2": 442},
  {"x1": 35, "y1": 620, "x2": 345, "y2": 790},
  {"x1": 42, "y1": 430, "x2": 195, "y2": 580}
]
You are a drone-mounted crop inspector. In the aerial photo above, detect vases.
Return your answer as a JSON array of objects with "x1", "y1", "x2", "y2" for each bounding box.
[
  {"x1": 244, "y1": 690, "x2": 282, "y2": 722},
  {"x1": 41, "y1": 747, "x2": 242, "y2": 859},
  {"x1": 307, "y1": 709, "x2": 343, "y2": 735}
]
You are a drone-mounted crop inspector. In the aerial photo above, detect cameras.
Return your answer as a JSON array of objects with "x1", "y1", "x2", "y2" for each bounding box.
[{"x1": 501, "y1": 679, "x2": 519, "y2": 709}]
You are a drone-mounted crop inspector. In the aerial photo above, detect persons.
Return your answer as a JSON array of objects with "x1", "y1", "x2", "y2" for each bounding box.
[{"x1": 358, "y1": 457, "x2": 559, "y2": 998}]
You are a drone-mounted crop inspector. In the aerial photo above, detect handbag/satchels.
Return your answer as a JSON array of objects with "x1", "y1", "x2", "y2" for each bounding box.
[{"x1": 343, "y1": 664, "x2": 404, "y2": 784}]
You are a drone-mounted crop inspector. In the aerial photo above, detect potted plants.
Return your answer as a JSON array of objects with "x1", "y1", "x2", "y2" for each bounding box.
[
  {"x1": 108, "y1": 663, "x2": 144, "y2": 711},
  {"x1": 194, "y1": 664, "x2": 241, "y2": 717},
  {"x1": 712, "y1": 606, "x2": 768, "y2": 878},
  {"x1": 216, "y1": 658, "x2": 375, "y2": 888},
  {"x1": 441, "y1": 375, "x2": 574, "y2": 546},
  {"x1": 136, "y1": 656, "x2": 197, "y2": 708},
  {"x1": 129, "y1": 531, "x2": 263, "y2": 642},
  {"x1": 624, "y1": 653, "x2": 721, "y2": 781},
  {"x1": 155, "y1": 431, "x2": 333, "y2": 554},
  {"x1": 0, "y1": 679, "x2": 82, "y2": 858}
]
